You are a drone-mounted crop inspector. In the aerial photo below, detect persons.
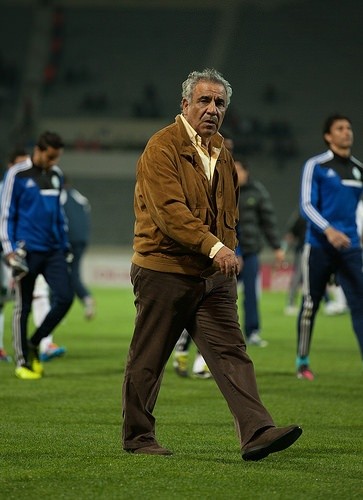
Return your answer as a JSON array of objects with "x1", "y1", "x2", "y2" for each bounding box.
[
  {"x1": 121, "y1": 69, "x2": 303, "y2": 461},
  {"x1": 0, "y1": 113, "x2": 363, "y2": 379}
]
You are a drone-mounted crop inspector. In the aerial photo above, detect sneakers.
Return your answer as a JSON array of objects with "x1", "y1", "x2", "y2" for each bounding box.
[{"x1": 40, "y1": 344, "x2": 65, "y2": 361}]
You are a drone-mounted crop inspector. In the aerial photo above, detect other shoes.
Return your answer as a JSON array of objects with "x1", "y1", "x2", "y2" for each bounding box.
[
  {"x1": 130, "y1": 446, "x2": 173, "y2": 455},
  {"x1": 298, "y1": 370, "x2": 314, "y2": 381},
  {"x1": 15, "y1": 365, "x2": 41, "y2": 379},
  {"x1": 31, "y1": 357, "x2": 45, "y2": 375},
  {"x1": 192, "y1": 368, "x2": 211, "y2": 379},
  {"x1": 242, "y1": 424, "x2": 302, "y2": 462},
  {"x1": 174, "y1": 353, "x2": 189, "y2": 376}
]
